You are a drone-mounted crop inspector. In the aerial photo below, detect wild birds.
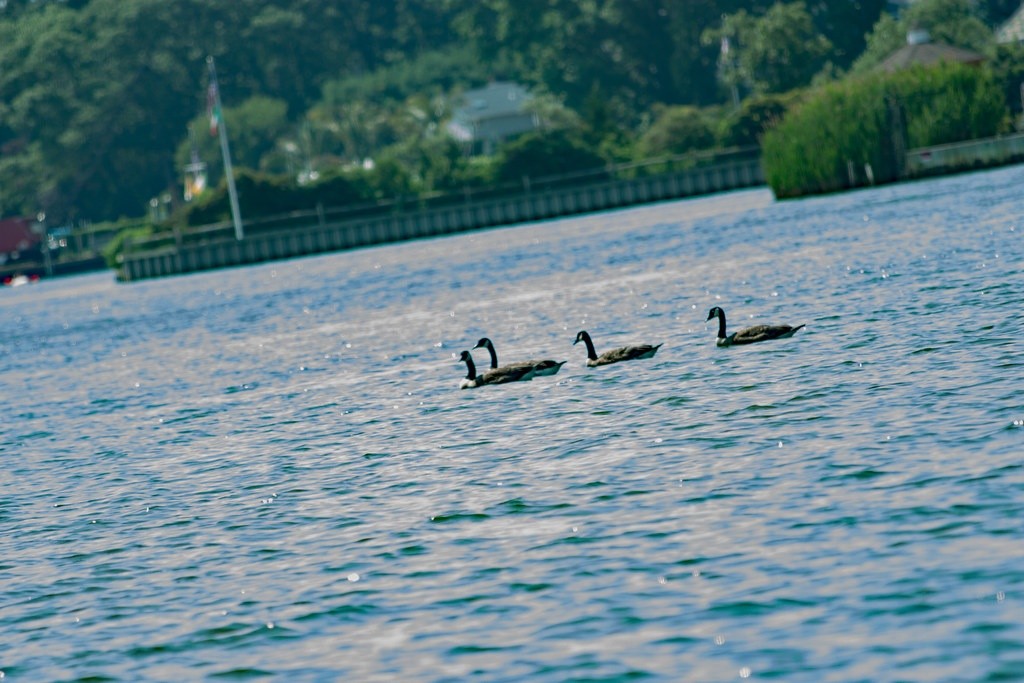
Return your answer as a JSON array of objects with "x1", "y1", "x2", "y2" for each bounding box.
[
  {"x1": 573, "y1": 330, "x2": 664, "y2": 368},
  {"x1": 457, "y1": 337, "x2": 568, "y2": 390},
  {"x1": 704, "y1": 306, "x2": 806, "y2": 348}
]
